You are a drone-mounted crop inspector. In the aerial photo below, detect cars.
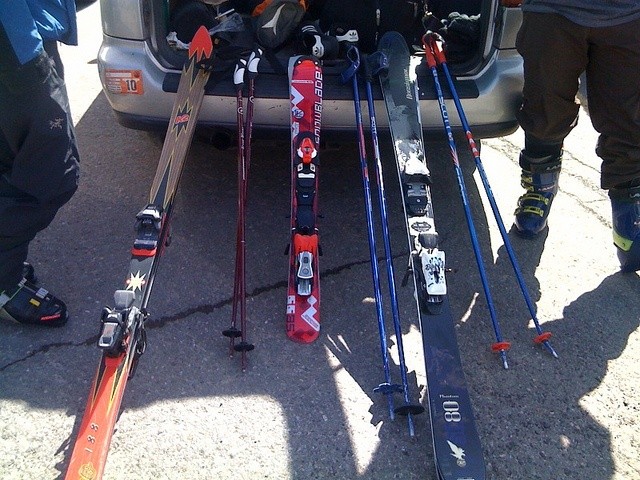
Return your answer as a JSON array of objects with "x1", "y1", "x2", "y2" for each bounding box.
[{"x1": 98, "y1": 0, "x2": 524, "y2": 151}]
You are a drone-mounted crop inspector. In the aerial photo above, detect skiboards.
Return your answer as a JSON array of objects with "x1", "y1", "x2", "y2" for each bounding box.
[
  {"x1": 64, "y1": 26, "x2": 214, "y2": 480},
  {"x1": 379, "y1": 33, "x2": 488, "y2": 480},
  {"x1": 286, "y1": 53, "x2": 325, "y2": 344}
]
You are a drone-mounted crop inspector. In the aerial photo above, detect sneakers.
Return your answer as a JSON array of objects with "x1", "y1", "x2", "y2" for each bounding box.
[
  {"x1": 323, "y1": 29, "x2": 359, "y2": 60},
  {"x1": 0, "y1": 276, "x2": 69, "y2": 327},
  {"x1": 612, "y1": 224, "x2": 640, "y2": 272},
  {"x1": 514, "y1": 190, "x2": 554, "y2": 233},
  {"x1": 299, "y1": 19, "x2": 339, "y2": 60}
]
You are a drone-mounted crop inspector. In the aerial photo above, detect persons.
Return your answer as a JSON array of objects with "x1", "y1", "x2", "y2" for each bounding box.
[
  {"x1": 0, "y1": 0, "x2": 100, "y2": 327},
  {"x1": 508, "y1": 0, "x2": 640, "y2": 273}
]
